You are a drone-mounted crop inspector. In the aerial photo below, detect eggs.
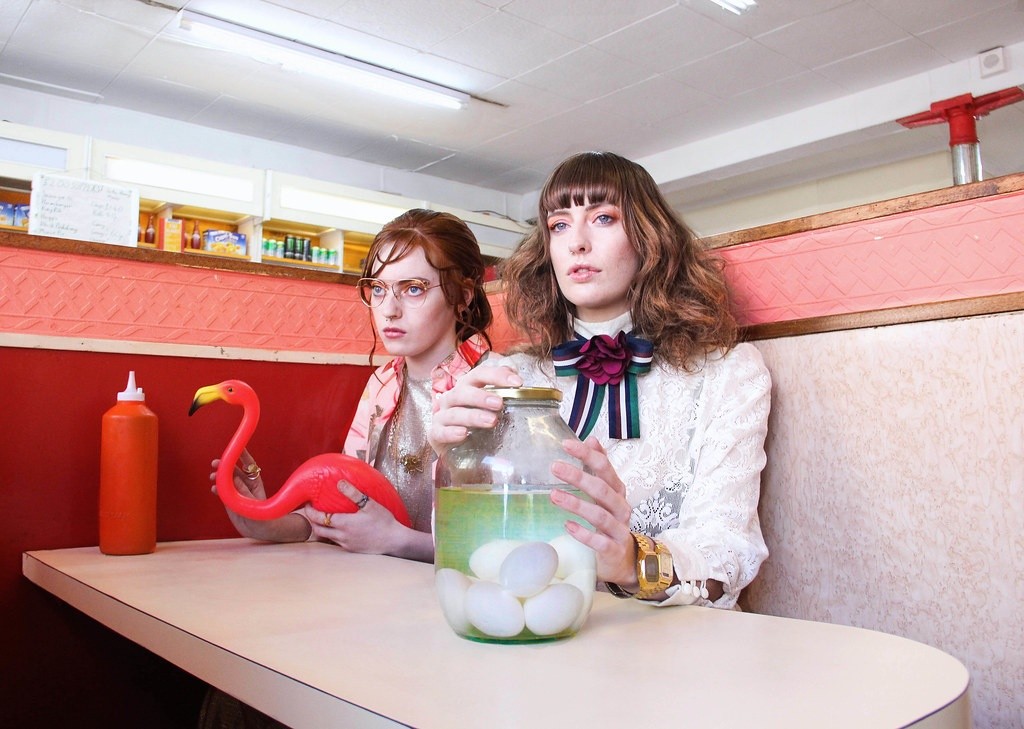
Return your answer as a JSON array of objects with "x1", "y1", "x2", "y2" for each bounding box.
[
  {"x1": 548, "y1": 536, "x2": 595, "y2": 577},
  {"x1": 500, "y1": 541, "x2": 558, "y2": 597},
  {"x1": 465, "y1": 580, "x2": 525, "y2": 637},
  {"x1": 522, "y1": 583, "x2": 584, "y2": 635},
  {"x1": 470, "y1": 540, "x2": 523, "y2": 581},
  {"x1": 562, "y1": 569, "x2": 596, "y2": 633},
  {"x1": 436, "y1": 567, "x2": 475, "y2": 632}
]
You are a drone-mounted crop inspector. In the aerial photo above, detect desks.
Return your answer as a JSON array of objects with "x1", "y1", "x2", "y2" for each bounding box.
[{"x1": 22, "y1": 539, "x2": 971, "y2": 729}]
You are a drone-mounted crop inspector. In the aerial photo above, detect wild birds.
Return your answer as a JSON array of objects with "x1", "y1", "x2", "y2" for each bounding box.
[{"x1": 187, "y1": 379, "x2": 413, "y2": 527}]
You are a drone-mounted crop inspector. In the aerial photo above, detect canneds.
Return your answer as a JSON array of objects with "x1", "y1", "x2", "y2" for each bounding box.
[{"x1": 261, "y1": 235, "x2": 337, "y2": 265}]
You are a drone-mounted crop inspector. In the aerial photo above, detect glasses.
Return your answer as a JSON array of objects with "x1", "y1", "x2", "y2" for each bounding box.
[{"x1": 356, "y1": 278, "x2": 463, "y2": 309}]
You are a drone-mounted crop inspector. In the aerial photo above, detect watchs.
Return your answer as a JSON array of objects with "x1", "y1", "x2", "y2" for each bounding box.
[{"x1": 605, "y1": 531, "x2": 673, "y2": 599}]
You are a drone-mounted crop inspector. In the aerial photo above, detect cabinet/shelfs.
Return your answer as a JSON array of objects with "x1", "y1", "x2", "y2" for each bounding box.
[{"x1": 0, "y1": 177, "x2": 377, "y2": 274}]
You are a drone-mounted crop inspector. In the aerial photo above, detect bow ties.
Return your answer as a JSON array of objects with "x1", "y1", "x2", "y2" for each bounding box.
[{"x1": 551, "y1": 328, "x2": 653, "y2": 441}]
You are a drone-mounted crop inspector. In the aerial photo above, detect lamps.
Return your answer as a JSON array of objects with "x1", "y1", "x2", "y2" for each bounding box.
[{"x1": 176, "y1": 9, "x2": 471, "y2": 110}]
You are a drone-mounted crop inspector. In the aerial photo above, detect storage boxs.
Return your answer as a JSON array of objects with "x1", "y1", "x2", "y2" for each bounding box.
[{"x1": 202, "y1": 229, "x2": 247, "y2": 257}]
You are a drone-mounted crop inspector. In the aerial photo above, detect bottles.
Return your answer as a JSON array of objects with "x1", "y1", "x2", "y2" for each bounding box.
[
  {"x1": 145, "y1": 215, "x2": 154, "y2": 242},
  {"x1": 435, "y1": 386, "x2": 597, "y2": 645},
  {"x1": 99, "y1": 369, "x2": 159, "y2": 557},
  {"x1": 138, "y1": 216, "x2": 141, "y2": 242},
  {"x1": 191, "y1": 220, "x2": 201, "y2": 249},
  {"x1": 183, "y1": 219, "x2": 188, "y2": 248}
]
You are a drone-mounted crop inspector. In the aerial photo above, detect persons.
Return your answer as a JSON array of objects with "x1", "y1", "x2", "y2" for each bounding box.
[
  {"x1": 426, "y1": 150, "x2": 772, "y2": 612},
  {"x1": 197, "y1": 208, "x2": 507, "y2": 728}
]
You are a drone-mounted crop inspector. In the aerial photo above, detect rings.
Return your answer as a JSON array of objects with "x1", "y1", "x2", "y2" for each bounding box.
[
  {"x1": 242, "y1": 462, "x2": 261, "y2": 480},
  {"x1": 436, "y1": 392, "x2": 444, "y2": 400},
  {"x1": 324, "y1": 513, "x2": 334, "y2": 528},
  {"x1": 356, "y1": 495, "x2": 368, "y2": 508}
]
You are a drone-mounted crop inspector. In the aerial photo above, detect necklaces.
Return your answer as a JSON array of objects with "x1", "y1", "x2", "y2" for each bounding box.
[{"x1": 388, "y1": 366, "x2": 431, "y2": 476}]
